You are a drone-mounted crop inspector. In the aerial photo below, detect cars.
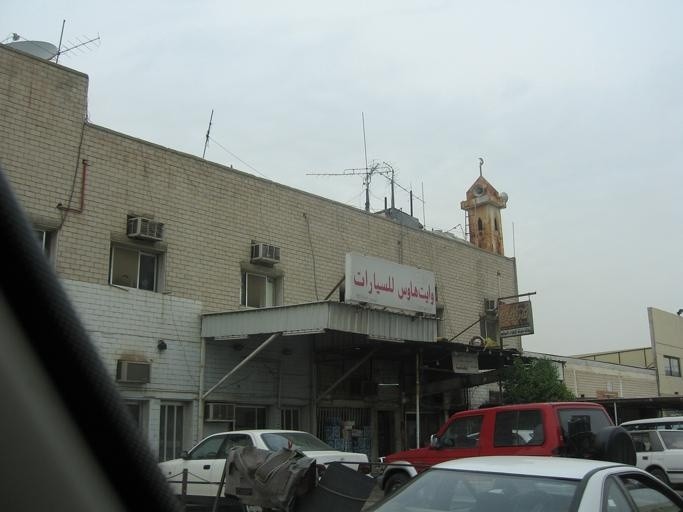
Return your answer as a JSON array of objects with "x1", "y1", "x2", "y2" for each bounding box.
[
  {"x1": 367, "y1": 454, "x2": 683, "y2": 511},
  {"x1": 157, "y1": 430, "x2": 372, "y2": 498},
  {"x1": 619, "y1": 417, "x2": 683, "y2": 486}
]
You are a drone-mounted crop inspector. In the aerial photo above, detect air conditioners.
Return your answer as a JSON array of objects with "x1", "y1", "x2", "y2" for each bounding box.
[
  {"x1": 485, "y1": 298, "x2": 506, "y2": 313},
  {"x1": 116, "y1": 360, "x2": 151, "y2": 385},
  {"x1": 250, "y1": 242, "x2": 280, "y2": 265},
  {"x1": 127, "y1": 216, "x2": 165, "y2": 241},
  {"x1": 204, "y1": 402, "x2": 235, "y2": 423}
]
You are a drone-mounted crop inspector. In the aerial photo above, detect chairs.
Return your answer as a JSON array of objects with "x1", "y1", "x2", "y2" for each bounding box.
[{"x1": 525, "y1": 424, "x2": 545, "y2": 444}]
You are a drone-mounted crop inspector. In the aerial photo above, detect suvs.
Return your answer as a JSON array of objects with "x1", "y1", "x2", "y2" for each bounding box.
[{"x1": 378, "y1": 402, "x2": 636, "y2": 496}]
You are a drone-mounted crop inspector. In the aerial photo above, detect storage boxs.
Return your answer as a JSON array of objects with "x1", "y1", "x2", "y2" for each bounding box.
[{"x1": 324, "y1": 425, "x2": 370, "y2": 458}]
